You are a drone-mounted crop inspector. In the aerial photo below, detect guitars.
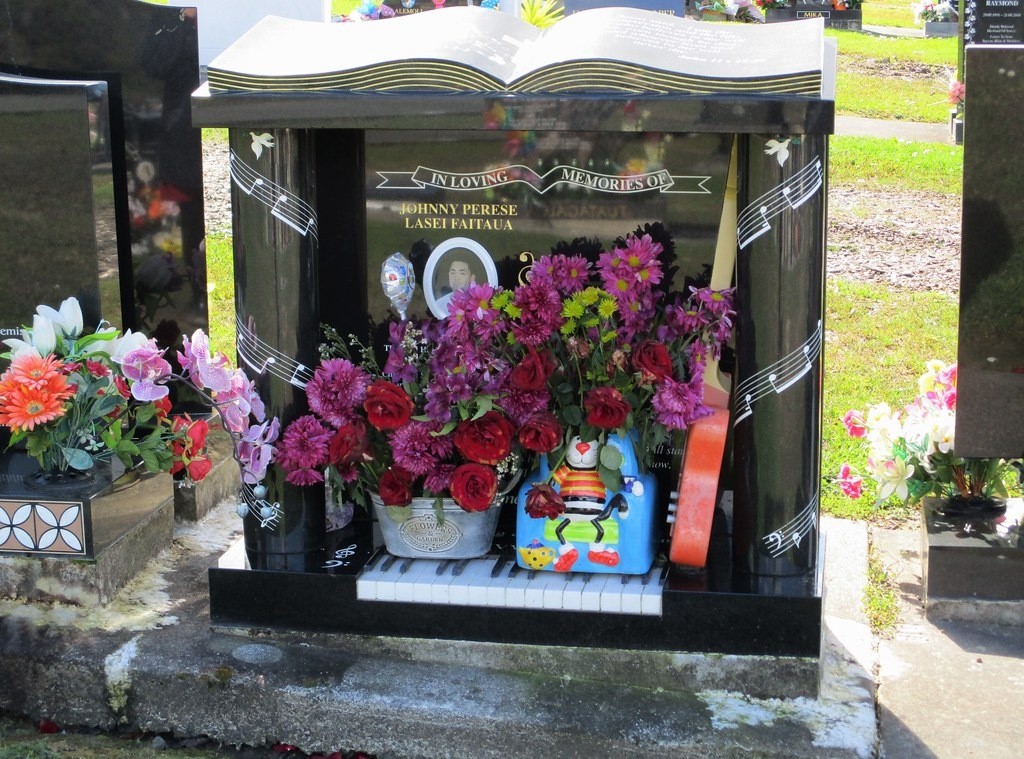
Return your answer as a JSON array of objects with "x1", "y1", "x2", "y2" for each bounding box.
[{"x1": 665, "y1": 133, "x2": 733, "y2": 568}]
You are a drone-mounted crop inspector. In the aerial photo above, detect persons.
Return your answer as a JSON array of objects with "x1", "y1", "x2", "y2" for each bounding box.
[{"x1": 435, "y1": 254, "x2": 477, "y2": 316}]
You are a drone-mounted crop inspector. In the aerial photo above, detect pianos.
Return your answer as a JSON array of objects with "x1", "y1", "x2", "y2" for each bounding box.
[{"x1": 206, "y1": 518, "x2": 824, "y2": 659}]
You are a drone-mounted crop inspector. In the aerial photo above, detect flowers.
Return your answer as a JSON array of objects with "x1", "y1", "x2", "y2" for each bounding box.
[
  {"x1": 839, "y1": 360, "x2": 1024, "y2": 506},
  {"x1": 0, "y1": 299, "x2": 281, "y2": 531},
  {"x1": 276, "y1": 285, "x2": 546, "y2": 510},
  {"x1": 510, "y1": 237, "x2": 737, "y2": 519}
]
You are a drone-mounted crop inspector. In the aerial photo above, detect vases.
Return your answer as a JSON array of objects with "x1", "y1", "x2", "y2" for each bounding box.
[
  {"x1": 516, "y1": 429, "x2": 659, "y2": 576},
  {"x1": 936, "y1": 495, "x2": 1007, "y2": 521},
  {"x1": 367, "y1": 486, "x2": 500, "y2": 559}
]
